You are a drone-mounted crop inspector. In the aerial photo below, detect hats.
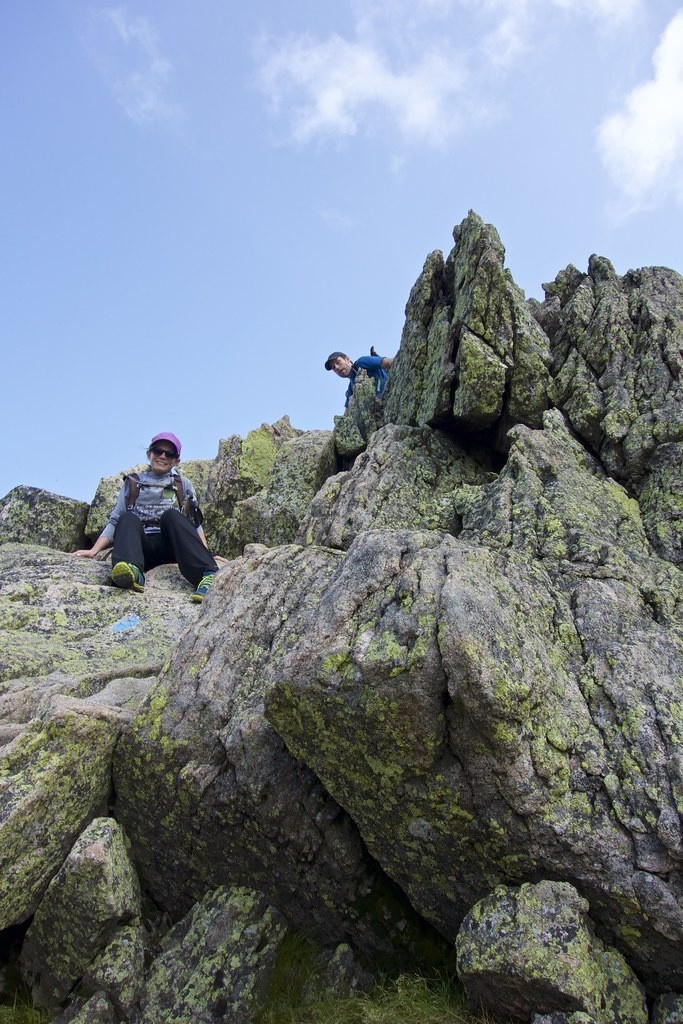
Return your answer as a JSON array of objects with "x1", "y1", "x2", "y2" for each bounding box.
[
  {"x1": 149, "y1": 430, "x2": 182, "y2": 455},
  {"x1": 324, "y1": 352, "x2": 347, "y2": 370}
]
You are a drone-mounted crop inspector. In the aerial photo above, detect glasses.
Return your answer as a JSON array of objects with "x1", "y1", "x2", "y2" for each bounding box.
[{"x1": 150, "y1": 446, "x2": 178, "y2": 460}]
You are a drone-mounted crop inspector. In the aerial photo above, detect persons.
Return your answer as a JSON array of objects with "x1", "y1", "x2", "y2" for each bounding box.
[
  {"x1": 72, "y1": 433, "x2": 219, "y2": 603},
  {"x1": 325, "y1": 352, "x2": 394, "y2": 417}
]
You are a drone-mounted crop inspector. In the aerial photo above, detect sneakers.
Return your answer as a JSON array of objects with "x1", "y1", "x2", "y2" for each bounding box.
[
  {"x1": 110, "y1": 561, "x2": 145, "y2": 593},
  {"x1": 192, "y1": 572, "x2": 217, "y2": 605}
]
